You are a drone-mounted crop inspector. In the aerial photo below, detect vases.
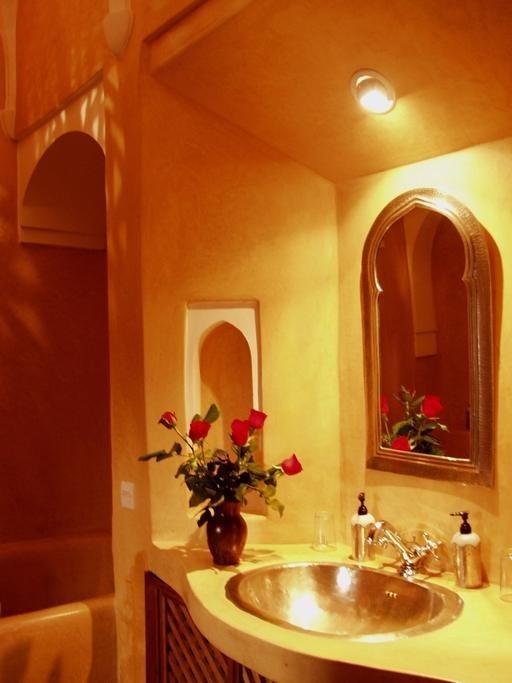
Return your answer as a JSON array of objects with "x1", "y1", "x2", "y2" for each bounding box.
[{"x1": 205, "y1": 498, "x2": 248, "y2": 566}]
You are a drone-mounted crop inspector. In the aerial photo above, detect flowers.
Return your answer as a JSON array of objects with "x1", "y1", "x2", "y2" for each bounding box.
[
  {"x1": 378, "y1": 386, "x2": 451, "y2": 456},
  {"x1": 138, "y1": 403, "x2": 304, "y2": 528}
]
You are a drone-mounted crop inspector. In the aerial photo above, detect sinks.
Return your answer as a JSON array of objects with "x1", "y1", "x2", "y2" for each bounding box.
[{"x1": 224, "y1": 561, "x2": 466, "y2": 642}]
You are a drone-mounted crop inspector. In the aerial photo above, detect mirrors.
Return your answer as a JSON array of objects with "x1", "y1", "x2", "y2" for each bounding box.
[{"x1": 359, "y1": 188, "x2": 497, "y2": 487}]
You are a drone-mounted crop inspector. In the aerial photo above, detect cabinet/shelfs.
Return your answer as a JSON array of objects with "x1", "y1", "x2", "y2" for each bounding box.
[{"x1": 141, "y1": 570, "x2": 275, "y2": 681}]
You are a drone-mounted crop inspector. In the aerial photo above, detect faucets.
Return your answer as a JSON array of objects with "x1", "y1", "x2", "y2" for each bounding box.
[{"x1": 365, "y1": 516, "x2": 421, "y2": 580}]
